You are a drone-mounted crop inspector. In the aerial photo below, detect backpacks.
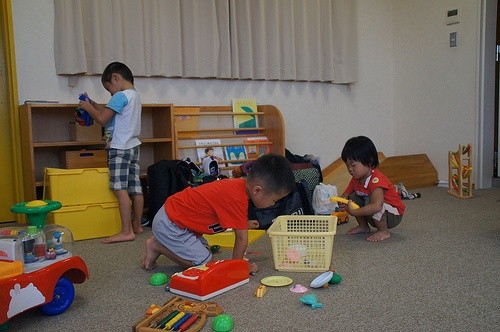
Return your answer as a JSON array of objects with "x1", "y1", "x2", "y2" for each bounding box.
[
  {"x1": 148, "y1": 159, "x2": 193, "y2": 225},
  {"x1": 203, "y1": 173, "x2": 315, "y2": 231}
]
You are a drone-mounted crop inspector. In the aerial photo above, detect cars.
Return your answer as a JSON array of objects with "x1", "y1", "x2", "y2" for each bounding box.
[
  {"x1": 330, "y1": 211, "x2": 349, "y2": 225},
  {"x1": 0, "y1": 201, "x2": 87, "y2": 332}
]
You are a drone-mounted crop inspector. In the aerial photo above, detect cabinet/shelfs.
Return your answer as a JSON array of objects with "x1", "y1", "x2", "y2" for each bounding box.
[
  {"x1": 173, "y1": 104, "x2": 285, "y2": 173},
  {"x1": 19, "y1": 104, "x2": 175, "y2": 223}
]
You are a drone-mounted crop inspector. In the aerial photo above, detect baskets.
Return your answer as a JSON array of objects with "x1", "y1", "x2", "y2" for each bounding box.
[{"x1": 267, "y1": 215, "x2": 339, "y2": 274}]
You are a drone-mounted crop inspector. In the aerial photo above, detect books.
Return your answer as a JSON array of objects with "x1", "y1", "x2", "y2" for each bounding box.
[
  {"x1": 232, "y1": 98, "x2": 260, "y2": 134},
  {"x1": 196, "y1": 136, "x2": 268, "y2": 176}
]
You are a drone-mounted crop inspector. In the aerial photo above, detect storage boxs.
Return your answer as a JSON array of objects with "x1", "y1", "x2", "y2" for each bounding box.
[
  {"x1": 58, "y1": 151, "x2": 109, "y2": 169},
  {"x1": 67, "y1": 120, "x2": 102, "y2": 142},
  {"x1": 268, "y1": 214, "x2": 338, "y2": 272},
  {"x1": 41, "y1": 166, "x2": 118, "y2": 206},
  {"x1": 50, "y1": 204, "x2": 124, "y2": 241}
]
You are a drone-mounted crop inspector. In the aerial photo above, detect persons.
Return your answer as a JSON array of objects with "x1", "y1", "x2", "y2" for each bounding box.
[
  {"x1": 77, "y1": 62, "x2": 147, "y2": 243},
  {"x1": 142, "y1": 154, "x2": 295, "y2": 273},
  {"x1": 337, "y1": 136, "x2": 404, "y2": 241}
]
